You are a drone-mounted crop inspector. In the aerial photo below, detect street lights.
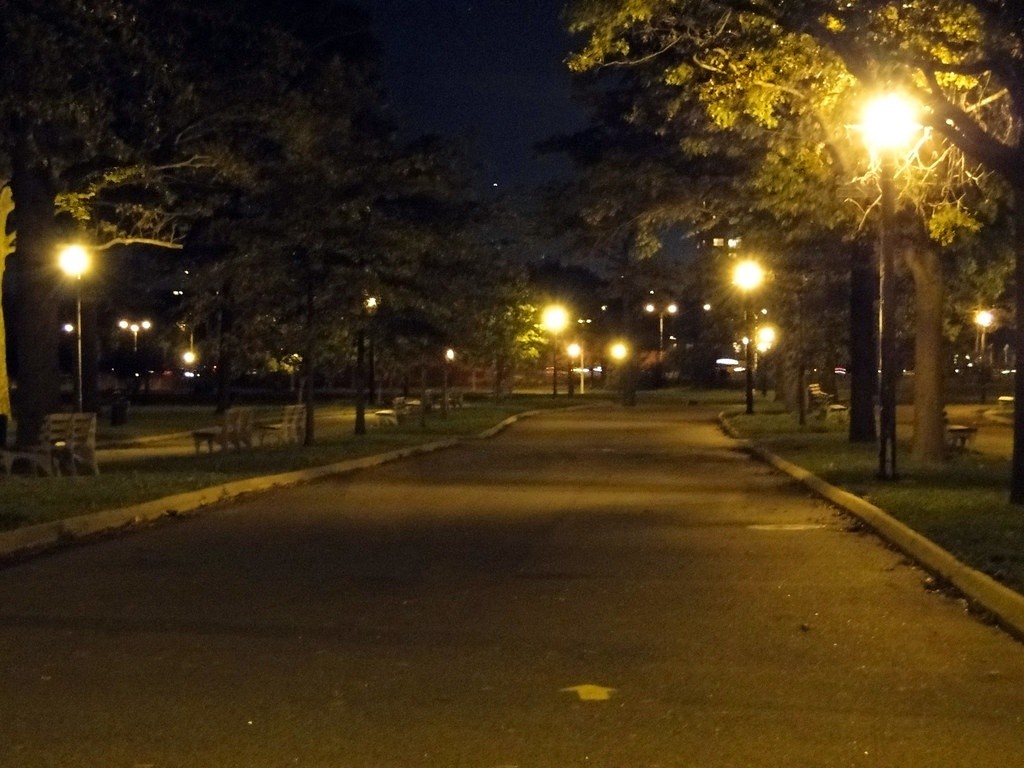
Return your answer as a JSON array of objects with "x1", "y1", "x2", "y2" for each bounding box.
[
  {"x1": 58, "y1": 245, "x2": 92, "y2": 441},
  {"x1": 976, "y1": 310, "x2": 994, "y2": 353},
  {"x1": 545, "y1": 307, "x2": 565, "y2": 400},
  {"x1": 735, "y1": 259, "x2": 763, "y2": 414},
  {"x1": 861, "y1": 88, "x2": 920, "y2": 476},
  {"x1": 659, "y1": 305, "x2": 678, "y2": 351}
]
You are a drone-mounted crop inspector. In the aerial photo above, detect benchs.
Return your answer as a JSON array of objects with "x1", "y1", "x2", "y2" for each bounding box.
[
  {"x1": 407, "y1": 389, "x2": 436, "y2": 417},
  {"x1": 191, "y1": 405, "x2": 273, "y2": 458},
  {"x1": 260, "y1": 405, "x2": 306, "y2": 447},
  {"x1": 439, "y1": 387, "x2": 464, "y2": 410},
  {"x1": 942, "y1": 399, "x2": 977, "y2": 450},
  {"x1": 821, "y1": 395, "x2": 847, "y2": 419},
  {"x1": 999, "y1": 396, "x2": 1014, "y2": 412},
  {"x1": 6, "y1": 413, "x2": 100, "y2": 493},
  {"x1": 809, "y1": 383, "x2": 834, "y2": 406},
  {"x1": 375, "y1": 397, "x2": 407, "y2": 428}
]
